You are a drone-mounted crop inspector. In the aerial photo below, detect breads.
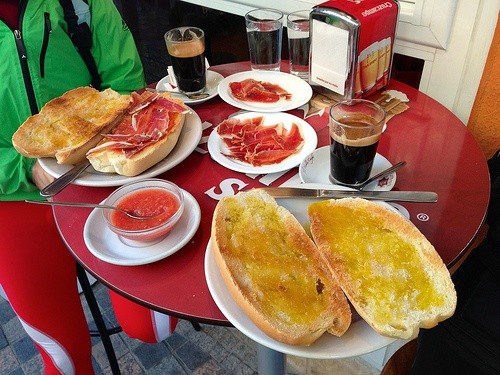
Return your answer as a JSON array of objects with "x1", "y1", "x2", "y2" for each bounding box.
[
  {"x1": 210, "y1": 188, "x2": 351, "y2": 347},
  {"x1": 12, "y1": 85, "x2": 185, "y2": 176},
  {"x1": 308, "y1": 198, "x2": 457, "y2": 340}
]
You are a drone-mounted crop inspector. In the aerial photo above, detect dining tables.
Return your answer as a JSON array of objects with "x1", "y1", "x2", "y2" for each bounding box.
[{"x1": 50, "y1": 56, "x2": 491, "y2": 375}]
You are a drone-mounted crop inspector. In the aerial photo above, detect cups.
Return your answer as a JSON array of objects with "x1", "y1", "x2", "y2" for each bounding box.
[
  {"x1": 287, "y1": 10, "x2": 312, "y2": 82},
  {"x1": 328, "y1": 98, "x2": 387, "y2": 189},
  {"x1": 164, "y1": 26, "x2": 206, "y2": 94},
  {"x1": 245, "y1": 8, "x2": 284, "y2": 72},
  {"x1": 355, "y1": 37, "x2": 392, "y2": 93}
]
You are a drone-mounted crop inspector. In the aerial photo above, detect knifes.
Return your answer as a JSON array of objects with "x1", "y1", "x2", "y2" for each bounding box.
[{"x1": 255, "y1": 186, "x2": 439, "y2": 202}]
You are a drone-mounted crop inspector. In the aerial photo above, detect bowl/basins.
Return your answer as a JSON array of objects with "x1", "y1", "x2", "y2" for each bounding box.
[{"x1": 102, "y1": 178, "x2": 185, "y2": 242}]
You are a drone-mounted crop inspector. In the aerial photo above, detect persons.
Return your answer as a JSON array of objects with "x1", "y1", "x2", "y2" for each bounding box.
[{"x1": 0, "y1": 0, "x2": 177, "y2": 375}]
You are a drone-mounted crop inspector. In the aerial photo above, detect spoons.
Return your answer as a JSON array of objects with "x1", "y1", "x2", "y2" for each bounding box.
[
  {"x1": 145, "y1": 87, "x2": 211, "y2": 100},
  {"x1": 24, "y1": 199, "x2": 152, "y2": 219}
]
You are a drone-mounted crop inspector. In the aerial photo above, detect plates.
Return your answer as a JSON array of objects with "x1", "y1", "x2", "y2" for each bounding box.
[
  {"x1": 217, "y1": 70, "x2": 313, "y2": 113},
  {"x1": 204, "y1": 194, "x2": 399, "y2": 359},
  {"x1": 155, "y1": 70, "x2": 224, "y2": 104},
  {"x1": 38, "y1": 96, "x2": 203, "y2": 186},
  {"x1": 299, "y1": 146, "x2": 397, "y2": 192},
  {"x1": 207, "y1": 112, "x2": 318, "y2": 175},
  {"x1": 83, "y1": 187, "x2": 201, "y2": 266}
]
